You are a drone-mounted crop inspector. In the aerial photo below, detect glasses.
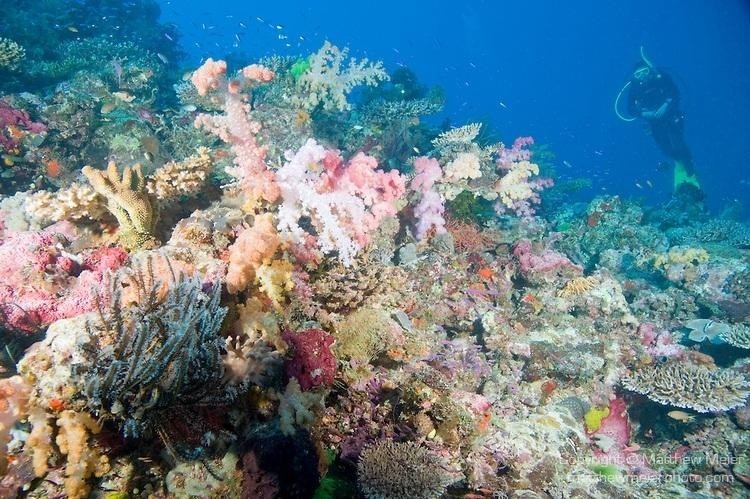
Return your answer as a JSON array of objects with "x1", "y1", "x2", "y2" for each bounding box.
[{"x1": 632, "y1": 67, "x2": 649, "y2": 78}]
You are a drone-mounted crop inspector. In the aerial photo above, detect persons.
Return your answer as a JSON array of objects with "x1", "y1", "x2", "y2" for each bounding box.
[
  {"x1": 360, "y1": 69, "x2": 428, "y2": 100},
  {"x1": 628, "y1": 59, "x2": 701, "y2": 195}
]
